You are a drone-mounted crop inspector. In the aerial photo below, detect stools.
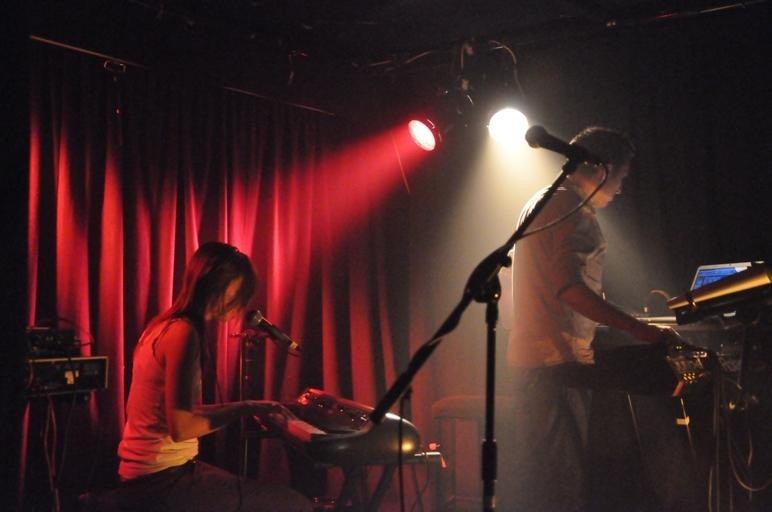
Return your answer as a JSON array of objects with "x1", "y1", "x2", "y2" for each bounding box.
[{"x1": 430, "y1": 394, "x2": 511, "y2": 509}]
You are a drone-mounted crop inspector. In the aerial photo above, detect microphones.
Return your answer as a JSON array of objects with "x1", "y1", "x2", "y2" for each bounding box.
[
  {"x1": 245, "y1": 310, "x2": 299, "y2": 351},
  {"x1": 525, "y1": 126, "x2": 601, "y2": 170}
]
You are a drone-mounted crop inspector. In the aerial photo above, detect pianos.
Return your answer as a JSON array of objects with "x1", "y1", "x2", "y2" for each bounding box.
[
  {"x1": 265, "y1": 388, "x2": 420, "y2": 466},
  {"x1": 591, "y1": 311, "x2": 761, "y2": 348}
]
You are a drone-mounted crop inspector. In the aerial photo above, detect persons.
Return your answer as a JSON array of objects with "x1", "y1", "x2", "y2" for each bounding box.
[
  {"x1": 510, "y1": 124, "x2": 689, "y2": 511},
  {"x1": 116, "y1": 238, "x2": 318, "y2": 511}
]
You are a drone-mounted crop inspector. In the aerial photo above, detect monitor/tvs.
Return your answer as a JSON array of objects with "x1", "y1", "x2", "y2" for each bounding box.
[{"x1": 688, "y1": 261, "x2": 754, "y2": 306}]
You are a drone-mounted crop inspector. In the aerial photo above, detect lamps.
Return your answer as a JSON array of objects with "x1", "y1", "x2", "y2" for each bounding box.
[{"x1": 404, "y1": 77, "x2": 479, "y2": 153}]
